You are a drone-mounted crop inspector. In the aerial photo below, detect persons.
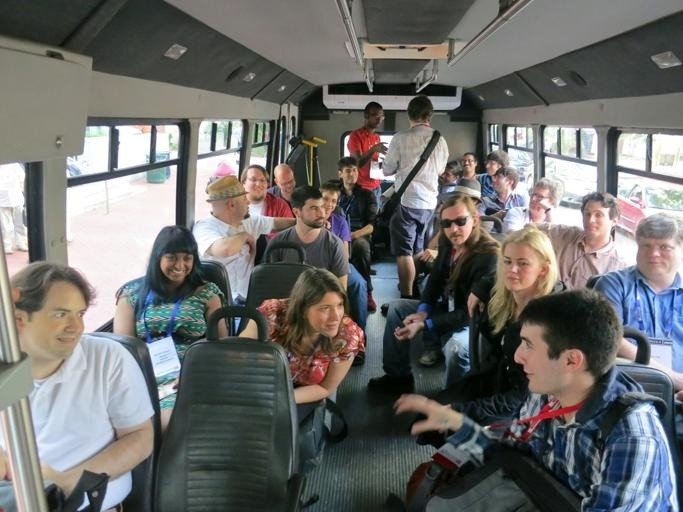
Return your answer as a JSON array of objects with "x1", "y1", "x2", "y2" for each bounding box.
[
  {"x1": 116, "y1": 151, "x2": 380, "y2": 423},
  {"x1": 348, "y1": 102, "x2": 388, "y2": 213},
  {"x1": 0, "y1": 262, "x2": 155, "y2": 512},
  {"x1": 369, "y1": 152, "x2": 683, "y2": 512},
  {"x1": 382, "y1": 96, "x2": 449, "y2": 312}
]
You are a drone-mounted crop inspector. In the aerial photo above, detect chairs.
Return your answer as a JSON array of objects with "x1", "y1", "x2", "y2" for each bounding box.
[{"x1": 84, "y1": 180, "x2": 677, "y2": 512}]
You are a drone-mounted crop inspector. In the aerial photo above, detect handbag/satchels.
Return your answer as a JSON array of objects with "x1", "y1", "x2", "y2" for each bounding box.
[
  {"x1": 375, "y1": 186, "x2": 402, "y2": 220},
  {"x1": 422, "y1": 441, "x2": 590, "y2": 512}
]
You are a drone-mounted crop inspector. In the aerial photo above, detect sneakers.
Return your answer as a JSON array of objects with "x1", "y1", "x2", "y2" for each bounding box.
[
  {"x1": 419, "y1": 348, "x2": 439, "y2": 366},
  {"x1": 368, "y1": 373, "x2": 416, "y2": 394},
  {"x1": 367, "y1": 295, "x2": 377, "y2": 312}
]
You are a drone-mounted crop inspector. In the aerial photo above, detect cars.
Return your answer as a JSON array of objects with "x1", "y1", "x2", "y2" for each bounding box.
[
  {"x1": 614, "y1": 183, "x2": 683, "y2": 241},
  {"x1": 66, "y1": 157, "x2": 93, "y2": 179},
  {"x1": 524, "y1": 155, "x2": 597, "y2": 208},
  {"x1": 492, "y1": 146, "x2": 532, "y2": 182}
]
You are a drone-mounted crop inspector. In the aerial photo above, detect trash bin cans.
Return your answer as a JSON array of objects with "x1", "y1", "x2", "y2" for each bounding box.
[{"x1": 145, "y1": 153, "x2": 170, "y2": 182}]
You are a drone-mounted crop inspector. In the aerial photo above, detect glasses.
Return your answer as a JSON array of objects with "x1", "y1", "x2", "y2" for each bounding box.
[
  {"x1": 440, "y1": 216, "x2": 470, "y2": 228},
  {"x1": 528, "y1": 187, "x2": 551, "y2": 201}
]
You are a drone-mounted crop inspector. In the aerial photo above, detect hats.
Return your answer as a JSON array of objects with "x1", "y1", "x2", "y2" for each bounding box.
[
  {"x1": 436, "y1": 177, "x2": 484, "y2": 204},
  {"x1": 205, "y1": 175, "x2": 250, "y2": 201}
]
